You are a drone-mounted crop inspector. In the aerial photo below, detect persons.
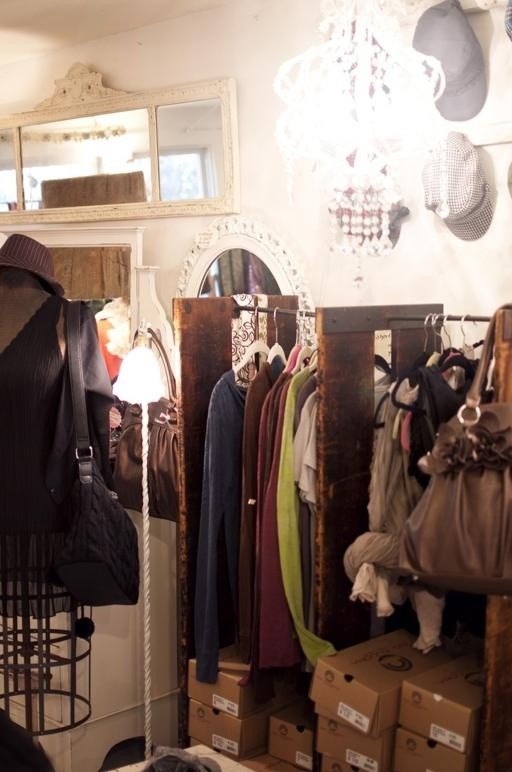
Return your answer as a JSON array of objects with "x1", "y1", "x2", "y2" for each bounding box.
[{"x1": 0, "y1": 234, "x2": 116, "y2": 529}]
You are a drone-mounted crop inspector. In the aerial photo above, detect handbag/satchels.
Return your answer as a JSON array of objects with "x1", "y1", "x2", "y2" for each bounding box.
[
  {"x1": 64, "y1": 299, "x2": 139, "y2": 607},
  {"x1": 114, "y1": 327, "x2": 179, "y2": 524},
  {"x1": 394, "y1": 300, "x2": 510, "y2": 595}
]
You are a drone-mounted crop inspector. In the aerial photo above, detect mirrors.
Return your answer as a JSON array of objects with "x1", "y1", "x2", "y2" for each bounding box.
[
  {"x1": 0, "y1": 63, "x2": 239, "y2": 228},
  {"x1": 174, "y1": 217, "x2": 310, "y2": 310}
]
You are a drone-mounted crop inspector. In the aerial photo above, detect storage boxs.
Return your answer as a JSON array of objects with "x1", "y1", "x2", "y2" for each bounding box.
[
  {"x1": 399, "y1": 656, "x2": 483, "y2": 756},
  {"x1": 189, "y1": 698, "x2": 269, "y2": 758},
  {"x1": 187, "y1": 643, "x2": 302, "y2": 718},
  {"x1": 269, "y1": 698, "x2": 315, "y2": 770},
  {"x1": 322, "y1": 755, "x2": 368, "y2": 772},
  {"x1": 318, "y1": 717, "x2": 396, "y2": 772},
  {"x1": 394, "y1": 727, "x2": 478, "y2": 772},
  {"x1": 309, "y1": 629, "x2": 454, "y2": 738}
]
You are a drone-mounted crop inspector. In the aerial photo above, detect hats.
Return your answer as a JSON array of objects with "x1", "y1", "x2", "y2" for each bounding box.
[
  {"x1": 325, "y1": 150, "x2": 407, "y2": 256},
  {"x1": 417, "y1": 132, "x2": 496, "y2": 243},
  {"x1": 0, "y1": 234, "x2": 67, "y2": 295},
  {"x1": 412, "y1": 0, "x2": 490, "y2": 123}
]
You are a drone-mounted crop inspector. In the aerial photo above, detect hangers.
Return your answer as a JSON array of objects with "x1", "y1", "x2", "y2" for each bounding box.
[
  {"x1": 416, "y1": 311, "x2": 477, "y2": 361},
  {"x1": 232, "y1": 305, "x2": 319, "y2": 375}
]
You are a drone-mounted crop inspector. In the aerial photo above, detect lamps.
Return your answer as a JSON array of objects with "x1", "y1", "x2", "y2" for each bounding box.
[{"x1": 111, "y1": 340, "x2": 171, "y2": 759}]
